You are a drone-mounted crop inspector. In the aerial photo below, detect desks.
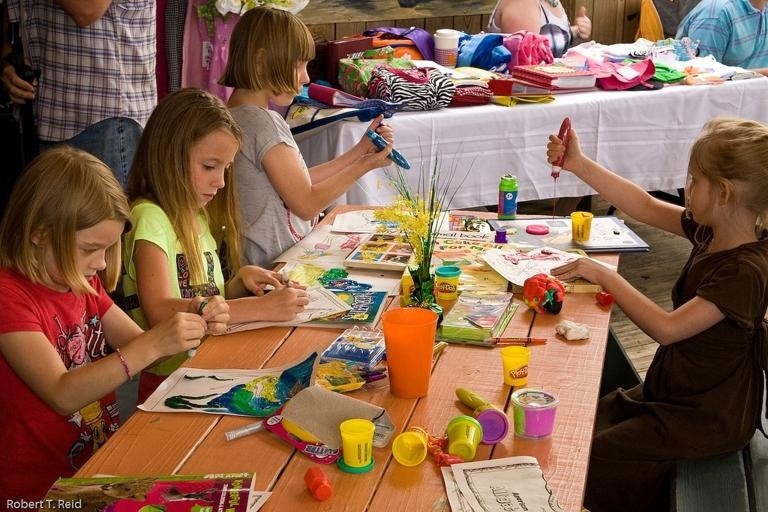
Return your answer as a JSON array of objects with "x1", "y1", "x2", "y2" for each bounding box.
[
  {"x1": 298, "y1": 76, "x2": 768, "y2": 215},
  {"x1": 70, "y1": 204, "x2": 624, "y2": 512}
]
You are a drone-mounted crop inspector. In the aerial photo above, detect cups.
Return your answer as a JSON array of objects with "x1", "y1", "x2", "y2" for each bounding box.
[
  {"x1": 379, "y1": 306, "x2": 440, "y2": 400},
  {"x1": 570, "y1": 210, "x2": 594, "y2": 242},
  {"x1": 433, "y1": 28, "x2": 460, "y2": 70},
  {"x1": 509, "y1": 387, "x2": 561, "y2": 441},
  {"x1": 339, "y1": 418, "x2": 376, "y2": 467},
  {"x1": 434, "y1": 265, "x2": 462, "y2": 301},
  {"x1": 391, "y1": 425, "x2": 430, "y2": 467},
  {"x1": 500, "y1": 345, "x2": 532, "y2": 386},
  {"x1": 447, "y1": 415, "x2": 484, "y2": 461}
]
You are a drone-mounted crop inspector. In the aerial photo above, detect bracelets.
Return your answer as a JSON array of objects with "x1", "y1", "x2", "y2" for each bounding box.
[{"x1": 112, "y1": 344, "x2": 136, "y2": 384}]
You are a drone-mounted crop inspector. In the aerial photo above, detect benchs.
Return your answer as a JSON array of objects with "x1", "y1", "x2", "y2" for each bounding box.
[{"x1": 609, "y1": 206, "x2": 768, "y2": 512}]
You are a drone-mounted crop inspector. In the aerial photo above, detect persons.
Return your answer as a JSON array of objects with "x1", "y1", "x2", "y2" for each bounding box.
[
  {"x1": 671, "y1": 0, "x2": 768, "y2": 78},
  {"x1": 224, "y1": 5, "x2": 397, "y2": 270},
  {"x1": 116, "y1": 85, "x2": 311, "y2": 417},
  {"x1": 544, "y1": 116, "x2": 768, "y2": 512},
  {"x1": 484, "y1": 0, "x2": 595, "y2": 59},
  {"x1": 1, "y1": 1, "x2": 161, "y2": 198},
  {"x1": 0, "y1": 144, "x2": 232, "y2": 512}
]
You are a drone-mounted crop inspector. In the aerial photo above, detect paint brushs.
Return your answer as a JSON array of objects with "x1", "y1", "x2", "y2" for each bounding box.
[
  {"x1": 224, "y1": 420, "x2": 266, "y2": 441},
  {"x1": 484, "y1": 337, "x2": 547, "y2": 345},
  {"x1": 455, "y1": 387, "x2": 487, "y2": 410},
  {"x1": 187, "y1": 301, "x2": 208, "y2": 361},
  {"x1": 283, "y1": 269, "x2": 289, "y2": 287},
  {"x1": 551, "y1": 117, "x2": 571, "y2": 178},
  {"x1": 365, "y1": 129, "x2": 411, "y2": 170},
  {"x1": 359, "y1": 367, "x2": 387, "y2": 383}
]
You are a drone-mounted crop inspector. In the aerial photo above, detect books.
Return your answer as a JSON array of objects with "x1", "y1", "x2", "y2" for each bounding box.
[
  {"x1": 344, "y1": 227, "x2": 415, "y2": 272},
  {"x1": 37, "y1": 470, "x2": 258, "y2": 512},
  {"x1": 510, "y1": 58, "x2": 599, "y2": 95},
  {"x1": 485, "y1": 212, "x2": 652, "y2": 254},
  {"x1": 237, "y1": 287, "x2": 389, "y2": 331}
]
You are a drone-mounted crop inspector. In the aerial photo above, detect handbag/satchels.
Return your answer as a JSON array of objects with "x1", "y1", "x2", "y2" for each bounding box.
[
  {"x1": 338, "y1": 46, "x2": 417, "y2": 98},
  {"x1": 368, "y1": 61, "x2": 456, "y2": 114}
]
